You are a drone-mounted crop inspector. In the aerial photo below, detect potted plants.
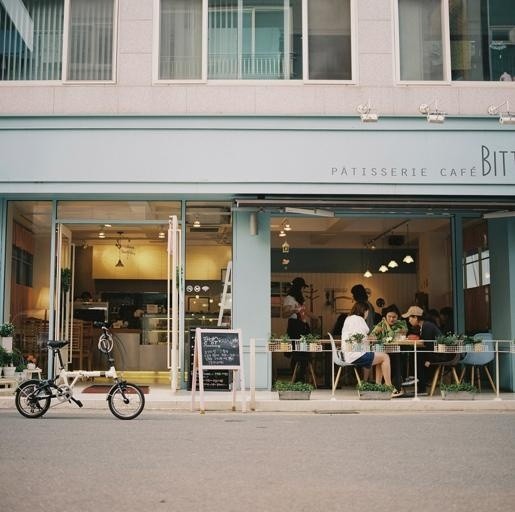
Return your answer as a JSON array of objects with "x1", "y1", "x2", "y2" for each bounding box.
[
  {"x1": 433, "y1": 331, "x2": 483, "y2": 352},
  {"x1": 273, "y1": 379, "x2": 315, "y2": 400},
  {"x1": 345, "y1": 332, "x2": 365, "y2": 352},
  {"x1": 356, "y1": 380, "x2": 395, "y2": 400},
  {"x1": 0, "y1": 322, "x2": 28, "y2": 380},
  {"x1": 267, "y1": 332, "x2": 323, "y2": 352},
  {"x1": 368, "y1": 319, "x2": 408, "y2": 352}
]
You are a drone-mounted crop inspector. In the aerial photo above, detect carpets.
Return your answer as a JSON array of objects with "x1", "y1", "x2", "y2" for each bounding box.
[{"x1": 81, "y1": 384, "x2": 149, "y2": 394}]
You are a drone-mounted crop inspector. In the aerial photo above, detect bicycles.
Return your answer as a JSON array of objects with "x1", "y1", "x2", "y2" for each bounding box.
[{"x1": 15, "y1": 326, "x2": 145, "y2": 420}]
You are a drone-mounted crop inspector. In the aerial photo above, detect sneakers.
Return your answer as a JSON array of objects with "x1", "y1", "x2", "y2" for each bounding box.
[{"x1": 393, "y1": 376, "x2": 419, "y2": 397}]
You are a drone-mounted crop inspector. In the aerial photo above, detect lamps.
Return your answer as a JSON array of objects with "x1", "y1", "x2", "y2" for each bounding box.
[
  {"x1": 279, "y1": 218, "x2": 291, "y2": 238},
  {"x1": 114, "y1": 231, "x2": 125, "y2": 267},
  {"x1": 363, "y1": 219, "x2": 414, "y2": 278}
]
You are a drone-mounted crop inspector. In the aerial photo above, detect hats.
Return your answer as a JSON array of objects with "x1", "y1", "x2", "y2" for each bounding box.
[
  {"x1": 292, "y1": 278, "x2": 309, "y2": 288},
  {"x1": 401, "y1": 305, "x2": 424, "y2": 318}
]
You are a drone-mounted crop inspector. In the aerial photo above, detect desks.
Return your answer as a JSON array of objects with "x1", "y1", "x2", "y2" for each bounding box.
[{"x1": 383, "y1": 339, "x2": 437, "y2": 400}]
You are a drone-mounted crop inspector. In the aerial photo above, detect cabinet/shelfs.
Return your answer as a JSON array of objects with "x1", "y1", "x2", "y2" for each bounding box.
[
  {"x1": 141, "y1": 313, "x2": 231, "y2": 345},
  {"x1": 271, "y1": 281, "x2": 295, "y2": 376}
]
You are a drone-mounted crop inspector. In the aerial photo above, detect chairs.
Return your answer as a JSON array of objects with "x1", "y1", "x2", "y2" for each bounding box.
[
  {"x1": 290, "y1": 328, "x2": 319, "y2": 390},
  {"x1": 327, "y1": 331, "x2": 362, "y2": 396},
  {"x1": 428, "y1": 332, "x2": 497, "y2": 400}
]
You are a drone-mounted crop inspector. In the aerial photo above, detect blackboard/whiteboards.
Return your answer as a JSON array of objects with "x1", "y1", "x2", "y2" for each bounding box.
[{"x1": 197, "y1": 328, "x2": 244, "y2": 370}]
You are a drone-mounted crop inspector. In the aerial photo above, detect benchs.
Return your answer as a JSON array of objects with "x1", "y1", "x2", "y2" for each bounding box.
[{"x1": 20, "y1": 318, "x2": 94, "y2": 370}]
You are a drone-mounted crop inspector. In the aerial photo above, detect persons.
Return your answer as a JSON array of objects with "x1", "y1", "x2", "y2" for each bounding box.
[
  {"x1": 281, "y1": 277, "x2": 310, "y2": 383},
  {"x1": 340, "y1": 284, "x2": 456, "y2": 398},
  {"x1": 126, "y1": 305, "x2": 149, "y2": 329}
]
